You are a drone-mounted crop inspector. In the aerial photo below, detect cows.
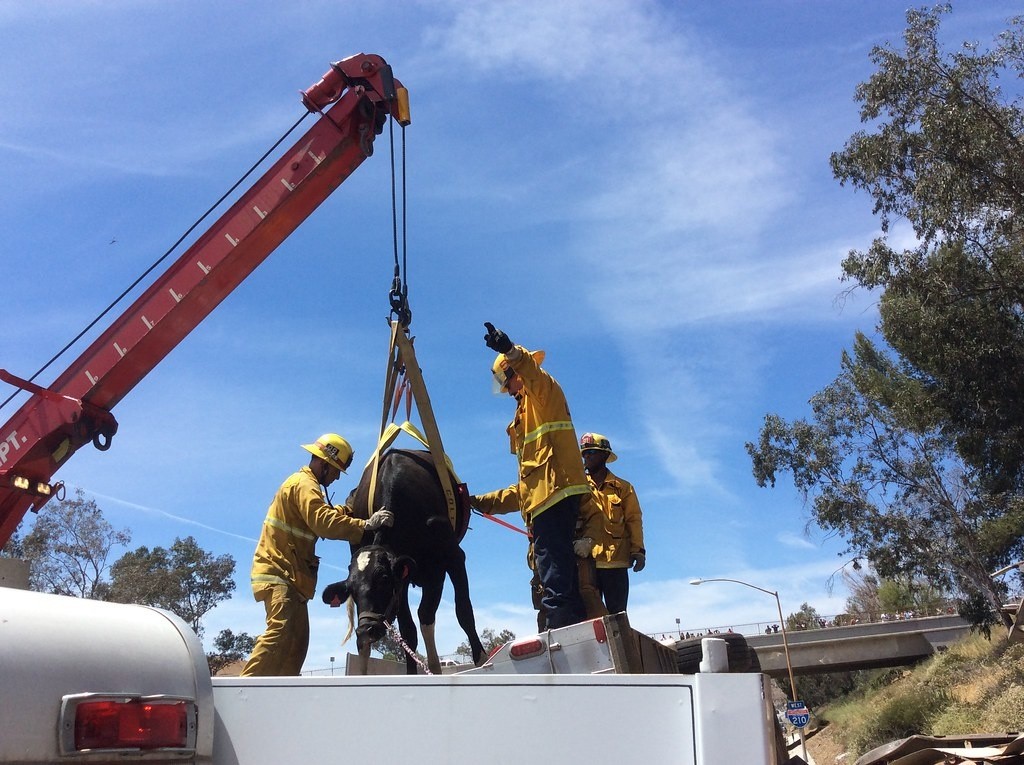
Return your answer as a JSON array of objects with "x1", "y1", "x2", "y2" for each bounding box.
[{"x1": 322, "y1": 447, "x2": 490, "y2": 675}]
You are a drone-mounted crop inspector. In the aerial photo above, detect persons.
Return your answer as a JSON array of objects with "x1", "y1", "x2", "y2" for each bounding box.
[
  {"x1": 236, "y1": 432, "x2": 394, "y2": 678},
  {"x1": 468, "y1": 321, "x2": 646, "y2": 633},
  {"x1": 658, "y1": 628, "x2": 735, "y2": 641},
  {"x1": 817, "y1": 608, "x2": 921, "y2": 629},
  {"x1": 794, "y1": 620, "x2": 802, "y2": 632},
  {"x1": 764, "y1": 623, "x2": 780, "y2": 634}
]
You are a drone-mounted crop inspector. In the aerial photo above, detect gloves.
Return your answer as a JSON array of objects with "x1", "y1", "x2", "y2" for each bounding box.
[
  {"x1": 573, "y1": 538, "x2": 593, "y2": 559},
  {"x1": 629, "y1": 552, "x2": 646, "y2": 572},
  {"x1": 483, "y1": 322, "x2": 514, "y2": 355},
  {"x1": 364, "y1": 505, "x2": 394, "y2": 530}
]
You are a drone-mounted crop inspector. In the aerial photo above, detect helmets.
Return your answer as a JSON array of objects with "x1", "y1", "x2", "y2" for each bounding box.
[
  {"x1": 579, "y1": 432, "x2": 618, "y2": 464},
  {"x1": 300, "y1": 432, "x2": 355, "y2": 475},
  {"x1": 492, "y1": 345, "x2": 545, "y2": 393}
]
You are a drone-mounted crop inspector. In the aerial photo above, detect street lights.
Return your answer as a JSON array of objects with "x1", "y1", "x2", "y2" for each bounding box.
[{"x1": 691, "y1": 577, "x2": 807, "y2": 762}]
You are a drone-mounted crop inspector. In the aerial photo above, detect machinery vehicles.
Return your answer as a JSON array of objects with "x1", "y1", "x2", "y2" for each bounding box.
[{"x1": 0, "y1": 54, "x2": 410, "y2": 551}]
